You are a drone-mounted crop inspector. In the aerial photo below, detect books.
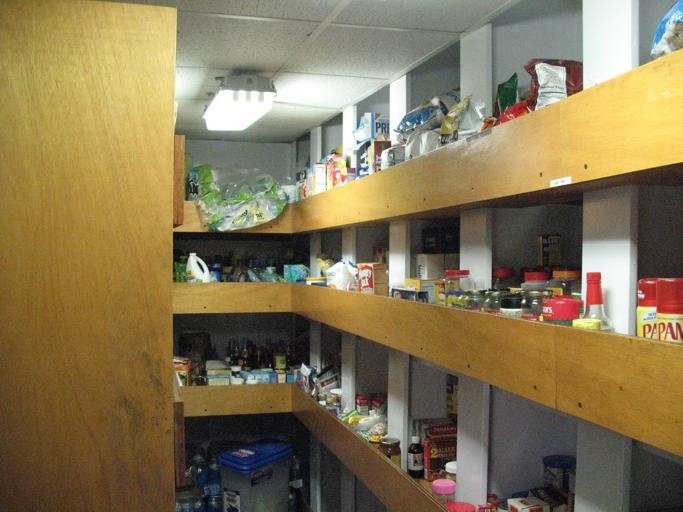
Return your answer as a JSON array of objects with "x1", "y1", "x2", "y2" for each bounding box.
[{"x1": 205, "y1": 358, "x2": 230, "y2": 387}]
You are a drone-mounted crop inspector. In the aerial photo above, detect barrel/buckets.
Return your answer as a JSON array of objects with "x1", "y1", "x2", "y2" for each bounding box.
[{"x1": 185, "y1": 252, "x2": 211, "y2": 283}]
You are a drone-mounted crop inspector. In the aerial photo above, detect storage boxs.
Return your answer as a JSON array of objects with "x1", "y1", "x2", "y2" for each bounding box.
[{"x1": 216, "y1": 436, "x2": 295, "y2": 512}]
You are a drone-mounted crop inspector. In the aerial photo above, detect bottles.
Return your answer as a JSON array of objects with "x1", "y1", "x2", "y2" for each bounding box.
[
  {"x1": 187, "y1": 169, "x2": 198, "y2": 200},
  {"x1": 315, "y1": 388, "x2": 341, "y2": 417},
  {"x1": 226, "y1": 333, "x2": 309, "y2": 373},
  {"x1": 204, "y1": 247, "x2": 307, "y2": 283},
  {"x1": 430, "y1": 461, "x2": 475, "y2": 512},
  {"x1": 438, "y1": 268, "x2": 613, "y2": 334},
  {"x1": 407, "y1": 435, "x2": 424, "y2": 478},
  {"x1": 172, "y1": 437, "x2": 221, "y2": 512},
  {"x1": 637, "y1": 277, "x2": 656, "y2": 339},
  {"x1": 289, "y1": 446, "x2": 310, "y2": 511},
  {"x1": 654, "y1": 276, "x2": 681, "y2": 341}
]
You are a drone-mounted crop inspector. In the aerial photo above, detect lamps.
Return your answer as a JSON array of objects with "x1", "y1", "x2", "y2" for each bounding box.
[{"x1": 200, "y1": 71, "x2": 277, "y2": 134}]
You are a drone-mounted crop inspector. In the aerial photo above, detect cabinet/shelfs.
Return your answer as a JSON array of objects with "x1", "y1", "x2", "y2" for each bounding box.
[{"x1": 171, "y1": 0, "x2": 681, "y2": 512}]
